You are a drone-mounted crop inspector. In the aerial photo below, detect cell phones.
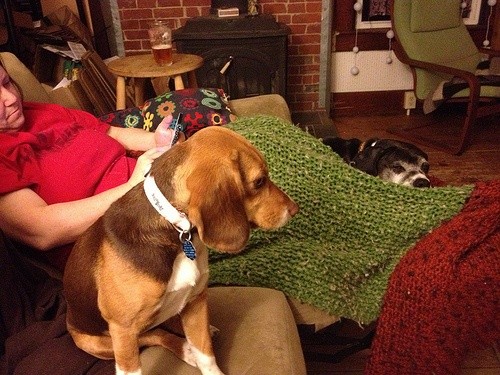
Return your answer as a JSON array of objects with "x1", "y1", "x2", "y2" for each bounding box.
[{"x1": 170, "y1": 113, "x2": 183, "y2": 146}]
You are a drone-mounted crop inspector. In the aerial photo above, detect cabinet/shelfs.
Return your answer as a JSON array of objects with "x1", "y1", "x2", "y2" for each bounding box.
[{"x1": 172, "y1": 15, "x2": 291, "y2": 100}]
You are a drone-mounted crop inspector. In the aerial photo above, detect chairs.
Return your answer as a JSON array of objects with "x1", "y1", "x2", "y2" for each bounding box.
[{"x1": 390, "y1": 0, "x2": 500, "y2": 156}]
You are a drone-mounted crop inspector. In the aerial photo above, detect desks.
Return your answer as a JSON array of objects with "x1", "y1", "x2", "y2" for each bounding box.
[{"x1": 108, "y1": 53, "x2": 204, "y2": 110}]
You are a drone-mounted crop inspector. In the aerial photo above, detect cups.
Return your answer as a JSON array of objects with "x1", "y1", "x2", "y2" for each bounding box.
[{"x1": 149, "y1": 28, "x2": 173, "y2": 67}]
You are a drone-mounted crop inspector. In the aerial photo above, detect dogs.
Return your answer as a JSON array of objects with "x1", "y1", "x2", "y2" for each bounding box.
[
  {"x1": 50, "y1": 125, "x2": 300, "y2": 375},
  {"x1": 320, "y1": 137, "x2": 432, "y2": 190}
]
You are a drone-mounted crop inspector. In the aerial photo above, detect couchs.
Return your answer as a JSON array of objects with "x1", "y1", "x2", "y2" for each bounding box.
[{"x1": 0, "y1": 52, "x2": 308, "y2": 375}]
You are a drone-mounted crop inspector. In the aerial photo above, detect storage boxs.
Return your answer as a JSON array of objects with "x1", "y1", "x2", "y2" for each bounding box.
[{"x1": 48, "y1": 80, "x2": 98, "y2": 116}]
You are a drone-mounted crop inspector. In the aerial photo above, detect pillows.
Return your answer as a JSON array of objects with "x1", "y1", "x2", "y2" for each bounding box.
[{"x1": 95, "y1": 88, "x2": 237, "y2": 140}]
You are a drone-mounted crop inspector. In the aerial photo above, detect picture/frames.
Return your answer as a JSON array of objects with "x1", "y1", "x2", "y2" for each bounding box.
[{"x1": 355, "y1": 0, "x2": 482, "y2": 29}]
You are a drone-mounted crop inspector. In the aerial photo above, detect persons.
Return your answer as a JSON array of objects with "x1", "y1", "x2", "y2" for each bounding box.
[{"x1": 0, "y1": 52, "x2": 186, "y2": 251}]
